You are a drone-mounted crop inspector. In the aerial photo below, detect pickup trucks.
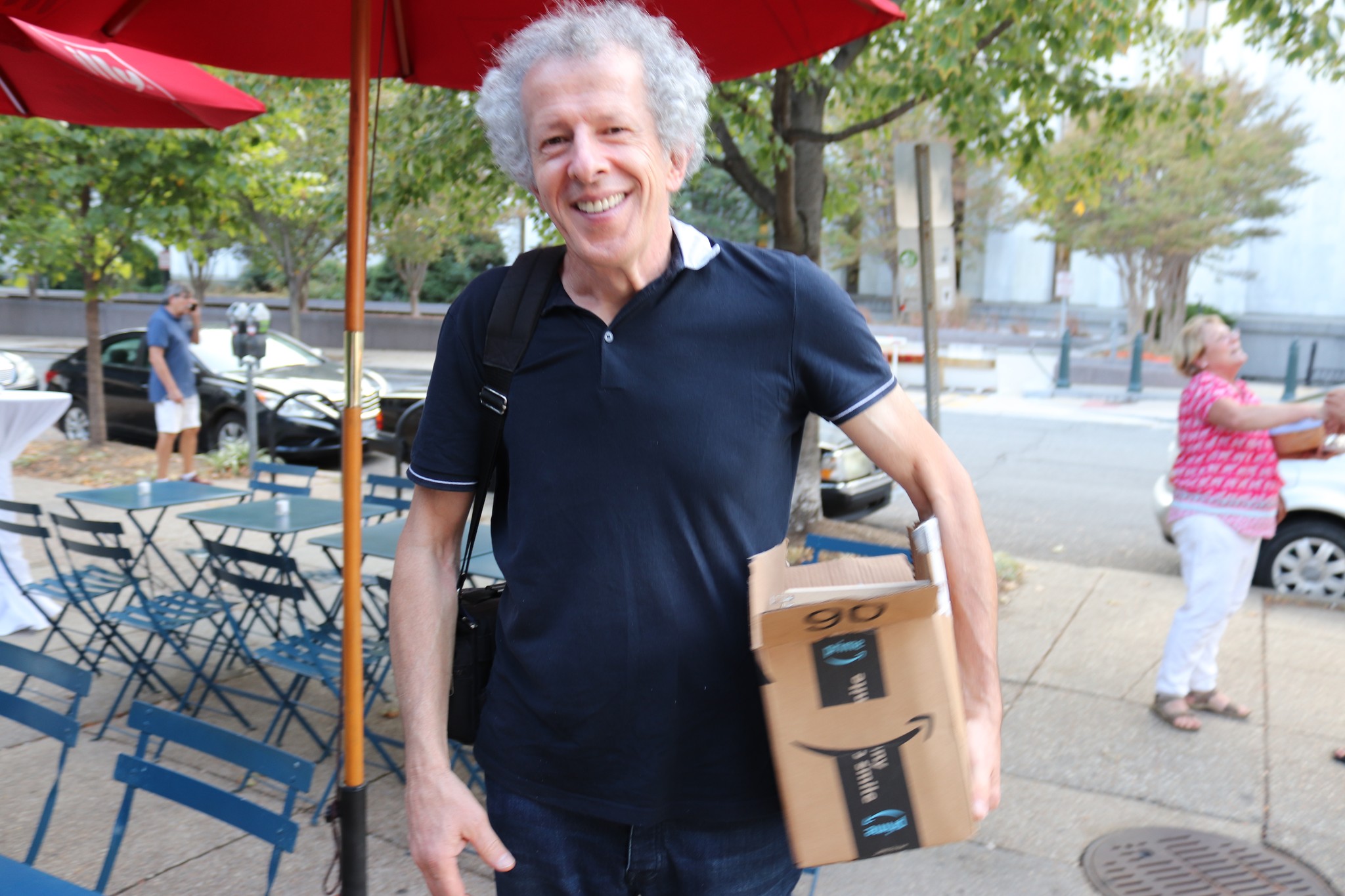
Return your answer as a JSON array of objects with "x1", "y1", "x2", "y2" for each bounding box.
[{"x1": 375, "y1": 387, "x2": 896, "y2": 523}]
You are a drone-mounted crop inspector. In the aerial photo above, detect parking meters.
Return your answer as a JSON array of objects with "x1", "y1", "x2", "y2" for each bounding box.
[{"x1": 225, "y1": 299, "x2": 273, "y2": 479}]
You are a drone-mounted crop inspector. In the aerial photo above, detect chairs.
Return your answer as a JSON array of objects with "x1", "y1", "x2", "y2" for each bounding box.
[{"x1": 0, "y1": 462, "x2": 414, "y2": 896}]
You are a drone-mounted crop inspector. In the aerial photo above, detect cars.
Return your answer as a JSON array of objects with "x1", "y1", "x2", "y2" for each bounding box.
[
  {"x1": 43, "y1": 321, "x2": 391, "y2": 471},
  {"x1": 1152, "y1": 385, "x2": 1345, "y2": 601}
]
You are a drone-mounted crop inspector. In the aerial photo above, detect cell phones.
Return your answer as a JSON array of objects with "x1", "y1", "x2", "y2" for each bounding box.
[{"x1": 186, "y1": 304, "x2": 196, "y2": 311}]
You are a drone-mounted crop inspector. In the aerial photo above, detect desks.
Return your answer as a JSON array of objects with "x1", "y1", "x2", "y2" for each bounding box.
[
  {"x1": 177, "y1": 497, "x2": 397, "y2": 637},
  {"x1": 308, "y1": 518, "x2": 493, "y2": 562},
  {"x1": 0, "y1": 389, "x2": 73, "y2": 635},
  {"x1": 54, "y1": 481, "x2": 251, "y2": 595},
  {"x1": 459, "y1": 553, "x2": 505, "y2": 580}
]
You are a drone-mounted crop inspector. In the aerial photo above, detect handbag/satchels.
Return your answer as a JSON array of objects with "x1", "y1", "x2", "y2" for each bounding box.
[{"x1": 447, "y1": 575, "x2": 513, "y2": 746}]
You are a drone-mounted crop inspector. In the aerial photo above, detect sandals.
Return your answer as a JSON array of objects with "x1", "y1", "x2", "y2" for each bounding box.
[
  {"x1": 1149, "y1": 692, "x2": 1202, "y2": 731},
  {"x1": 1186, "y1": 687, "x2": 1252, "y2": 720}
]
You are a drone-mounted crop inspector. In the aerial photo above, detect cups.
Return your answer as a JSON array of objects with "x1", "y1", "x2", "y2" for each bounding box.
[
  {"x1": 136, "y1": 473, "x2": 152, "y2": 496},
  {"x1": 275, "y1": 491, "x2": 289, "y2": 519}
]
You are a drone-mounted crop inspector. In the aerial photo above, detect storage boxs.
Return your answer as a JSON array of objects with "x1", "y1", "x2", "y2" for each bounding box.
[{"x1": 748, "y1": 517, "x2": 977, "y2": 870}]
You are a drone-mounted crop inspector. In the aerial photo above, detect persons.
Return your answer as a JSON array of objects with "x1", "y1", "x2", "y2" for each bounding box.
[
  {"x1": 388, "y1": 0, "x2": 1004, "y2": 896},
  {"x1": 145, "y1": 279, "x2": 211, "y2": 485},
  {"x1": 1148, "y1": 312, "x2": 1345, "y2": 761}
]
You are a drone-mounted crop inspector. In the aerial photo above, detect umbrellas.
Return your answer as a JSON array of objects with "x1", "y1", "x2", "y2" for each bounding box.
[
  {"x1": 0, "y1": 0, "x2": 905, "y2": 896},
  {"x1": 0, "y1": 13, "x2": 267, "y2": 134}
]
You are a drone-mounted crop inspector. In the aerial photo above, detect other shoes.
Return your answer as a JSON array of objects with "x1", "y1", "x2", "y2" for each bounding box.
[{"x1": 179, "y1": 474, "x2": 212, "y2": 486}]
